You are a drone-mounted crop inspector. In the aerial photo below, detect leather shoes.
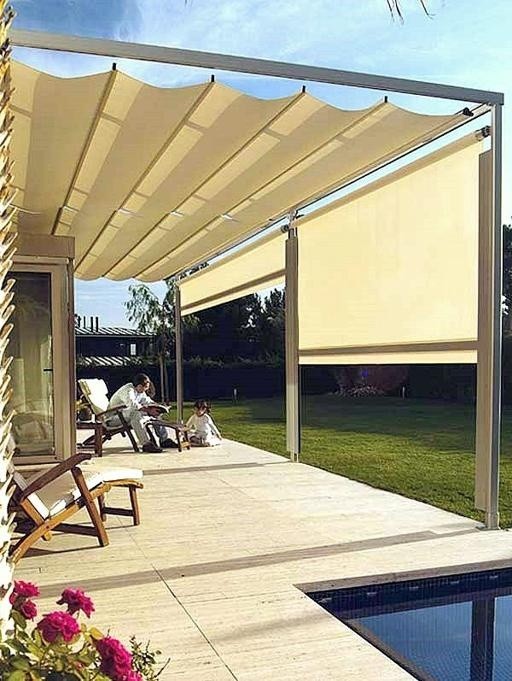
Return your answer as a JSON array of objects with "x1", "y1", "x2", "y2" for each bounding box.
[
  {"x1": 141, "y1": 440, "x2": 161, "y2": 454},
  {"x1": 160, "y1": 439, "x2": 180, "y2": 450}
]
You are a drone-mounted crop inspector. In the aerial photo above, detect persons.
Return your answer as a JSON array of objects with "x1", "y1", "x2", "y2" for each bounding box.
[
  {"x1": 183, "y1": 398, "x2": 223, "y2": 449},
  {"x1": 105, "y1": 374, "x2": 179, "y2": 455}
]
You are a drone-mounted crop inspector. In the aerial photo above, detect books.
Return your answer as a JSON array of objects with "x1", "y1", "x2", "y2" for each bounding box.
[{"x1": 146, "y1": 403, "x2": 173, "y2": 418}]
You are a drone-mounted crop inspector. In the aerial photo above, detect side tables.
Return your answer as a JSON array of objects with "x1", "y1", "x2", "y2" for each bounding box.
[{"x1": 76, "y1": 420, "x2": 103, "y2": 457}]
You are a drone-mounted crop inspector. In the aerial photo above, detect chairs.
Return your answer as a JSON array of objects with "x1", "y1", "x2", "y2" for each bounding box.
[
  {"x1": 8, "y1": 452, "x2": 143, "y2": 564},
  {"x1": 76, "y1": 377, "x2": 191, "y2": 452}
]
